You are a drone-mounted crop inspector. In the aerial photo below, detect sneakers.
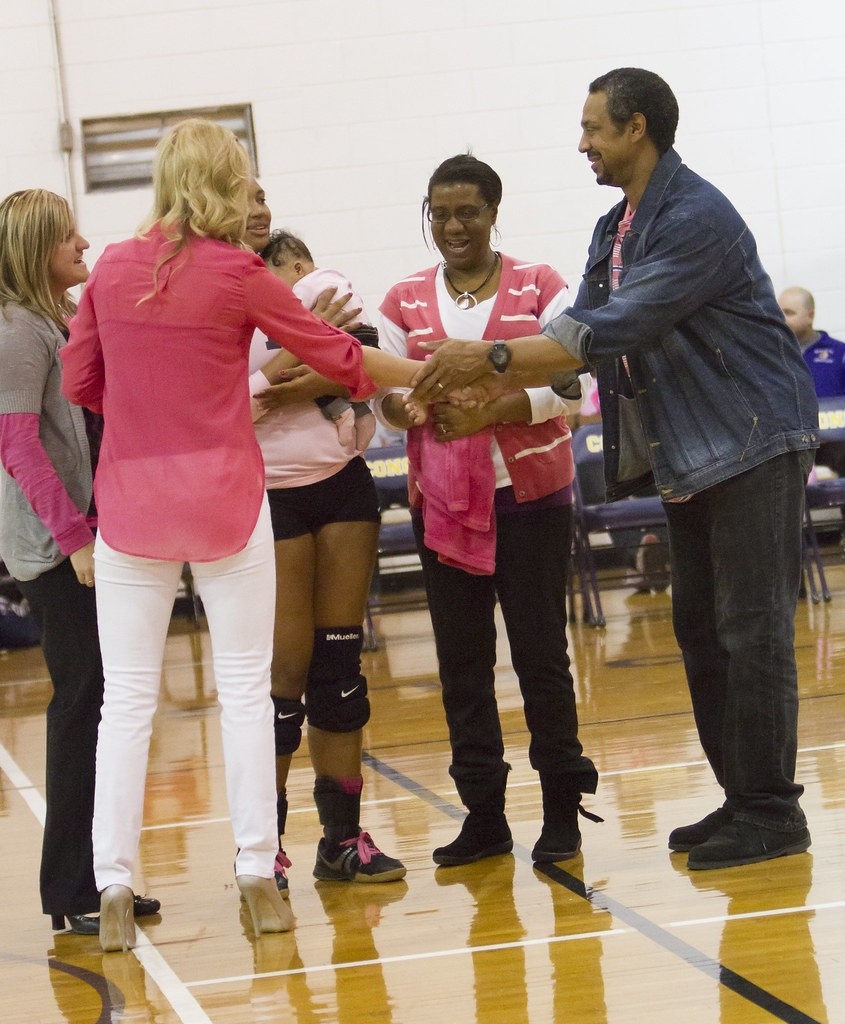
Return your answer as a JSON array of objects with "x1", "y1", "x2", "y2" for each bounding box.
[
  {"x1": 433, "y1": 814, "x2": 513, "y2": 864},
  {"x1": 687, "y1": 819, "x2": 811, "y2": 869},
  {"x1": 532, "y1": 804, "x2": 604, "y2": 860},
  {"x1": 668, "y1": 809, "x2": 734, "y2": 851},
  {"x1": 234, "y1": 848, "x2": 292, "y2": 901},
  {"x1": 313, "y1": 831, "x2": 406, "y2": 882}
]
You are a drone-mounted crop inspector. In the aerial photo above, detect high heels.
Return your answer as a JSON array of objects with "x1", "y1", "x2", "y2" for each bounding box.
[
  {"x1": 99, "y1": 884, "x2": 136, "y2": 952},
  {"x1": 237, "y1": 875, "x2": 293, "y2": 938},
  {"x1": 133, "y1": 894, "x2": 160, "y2": 914},
  {"x1": 51, "y1": 913, "x2": 100, "y2": 934}
]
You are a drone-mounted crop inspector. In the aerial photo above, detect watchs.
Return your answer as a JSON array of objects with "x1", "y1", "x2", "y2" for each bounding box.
[{"x1": 486, "y1": 338, "x2": 512, "y2": 375}]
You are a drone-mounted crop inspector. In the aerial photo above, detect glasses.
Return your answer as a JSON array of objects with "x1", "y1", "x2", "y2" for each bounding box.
[{"x1": 427, "y1": 202, "x2": 489, "y2": 223}]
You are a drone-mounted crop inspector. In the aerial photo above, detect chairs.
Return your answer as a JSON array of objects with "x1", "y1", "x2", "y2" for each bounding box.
[
  {"x1": 571, "y1": 424, "x2": 671, "y2": 628},
  {"x1": 803, "y1": 395, "x2": 845, "y2": 599},
  {"x1": 361, "y1": 445, "x2": 432, "y2": 650}
]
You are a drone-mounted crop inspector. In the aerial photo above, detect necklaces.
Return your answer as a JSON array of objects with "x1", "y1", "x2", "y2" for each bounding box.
[{"x1": 445, "y1": 252, "x2": 499, "y2": 311}]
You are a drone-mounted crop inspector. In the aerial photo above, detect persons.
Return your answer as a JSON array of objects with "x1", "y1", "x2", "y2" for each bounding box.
[
  {"x1": 0, "y1": 189, "x2": 162, "y2": 938},
  {"x1": 56, "y1": 115, "x2": 435, "y2": 957},
  {"x1": 776, "y1": 284, "x2": 845, "y2": 560},
  {"x1": 582, "y1": 365, "x2": 674, "y2": 593},
  {"x1": 376, "y1": 153, "x2": 604, "y2": 862},
  {"x1": 240, "y1": 224, "x2": 407, "y2": 898},
  {"x1": 402, "y1": 68, "x2": 813, "y2": 870}
]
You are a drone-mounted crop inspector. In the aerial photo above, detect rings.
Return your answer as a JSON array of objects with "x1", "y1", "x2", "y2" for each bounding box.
[
  {"x1": 437, "y1": 382, "x2": 444, "y2": 389},
  {"x1": 84, "y1": 579, "x2": 93, "y2": 583},
  {"x1": 440, "y1": 424, "x2": 447, "y2": 434}
]
